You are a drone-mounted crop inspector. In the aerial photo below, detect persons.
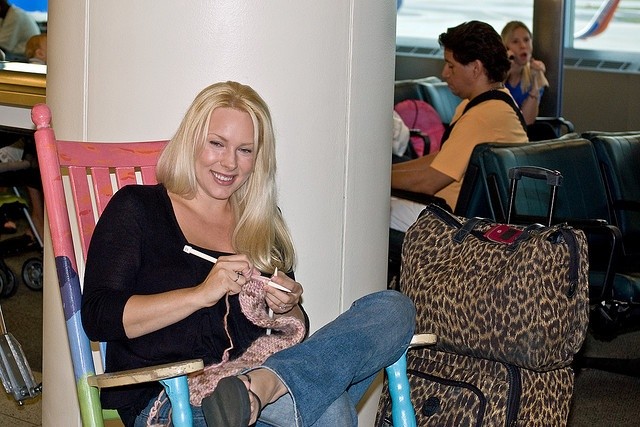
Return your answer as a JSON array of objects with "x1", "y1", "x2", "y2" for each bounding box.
[
  {"x1": 501, "y1": 22, "x2": 560, "y2": 142},
  {"x1": 390, "y1": 21, "x2": 530, "y2": 234},
  {"x1": 1, "y1": 186, "x2": 16, "y2": 236},
  {"x1": 23, "y1": 34, "x2": 48, "y2": 65},
  {"x1": 80, "y1": 80, "x2": 417, "y2": 426},
  {"x1": 0, "y1": 0, "x2": 40, "y2": 59},
  {"x1": 17, "y1": 130, "x2": 44, "y2": 251}
]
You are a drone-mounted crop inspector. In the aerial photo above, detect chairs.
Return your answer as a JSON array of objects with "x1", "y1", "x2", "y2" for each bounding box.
[
  {"x1": 388, "y1": 130, "x2": 582, "y2": 254},
  {"x1": 30, "y1": 102, "x2": 437, "y2": 426},
  {"x1": 582, "y1": 129, "x2": 637, "y2": 136},
  {"x1": 393, "y1": 76, "x2": 443, "y2": 106},
  {"x1": 594, "y1": 135, "x2": 639, "y2": 276},
  {"x1": 416, "y1": 80, "x2": 575, "y2": 142},
  {"x1": 481, "y1": 137, "x2": 639, "y2": 343}
]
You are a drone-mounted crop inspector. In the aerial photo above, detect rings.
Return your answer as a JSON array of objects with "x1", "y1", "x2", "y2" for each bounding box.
[{"x1": 234, "y1": 273, "x2": 240, "y2": 282}]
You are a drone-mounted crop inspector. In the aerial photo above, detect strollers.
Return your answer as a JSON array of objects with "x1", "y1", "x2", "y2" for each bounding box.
[{"x1": 1, "y1": 49, "x2": 44, "y2": 299}]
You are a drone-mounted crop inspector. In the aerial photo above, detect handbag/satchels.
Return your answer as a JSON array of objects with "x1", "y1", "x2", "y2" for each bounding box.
[{"x1": 400, "y1": 202, "x2": 590, "y2": 372}]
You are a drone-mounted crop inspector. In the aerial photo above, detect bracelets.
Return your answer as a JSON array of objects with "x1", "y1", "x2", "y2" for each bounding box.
[{"x1": 528, "y1": 93, "x2": 539, "y2": 101}]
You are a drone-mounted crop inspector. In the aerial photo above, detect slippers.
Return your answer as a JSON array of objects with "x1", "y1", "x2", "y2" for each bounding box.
[{"x1": 202, "y1": 374, "x2": 262, "y2": 427}]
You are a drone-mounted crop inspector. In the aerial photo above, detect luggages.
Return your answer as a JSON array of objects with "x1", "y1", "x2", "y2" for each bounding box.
[{"x1": 374, "y1": 165, "x2": 575, "y2": 427}]
